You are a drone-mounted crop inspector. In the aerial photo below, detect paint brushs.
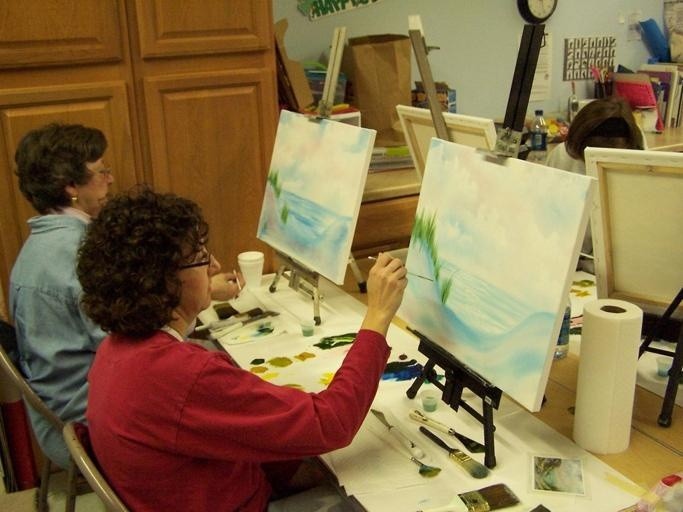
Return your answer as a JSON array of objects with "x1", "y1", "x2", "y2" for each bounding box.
[
  {"x1": 367, "y1": 255, "x2": 434, "y2": 282},
  {"x1": 365, "y1": 426, "x2": 442, "y2": 478},
  {"x1": 233, "y1": 270, "x2": 241, "y2": 290},
  {"x1": 371, "y1": 408, "x2": 424, "y2": 459},
  {"x1": 409, "y1": 410, "x2": 488, "y2": 453}
]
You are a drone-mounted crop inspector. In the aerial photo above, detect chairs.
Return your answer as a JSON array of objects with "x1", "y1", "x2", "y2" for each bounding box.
[
  {"x1": 61, "y1": 419, "x2": 133, "y2": 512},
  {"x1": 0, "y1": 345, "x2": 98, "y2": 511}
]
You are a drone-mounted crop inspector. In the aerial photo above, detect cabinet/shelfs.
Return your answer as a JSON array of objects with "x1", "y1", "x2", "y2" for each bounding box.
[{"x1": 0, "y1": 1, "x2": 286, "y2": 494}]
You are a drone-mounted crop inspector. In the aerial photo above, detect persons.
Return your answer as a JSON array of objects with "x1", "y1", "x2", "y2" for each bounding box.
[
  {"x1": 75, "y1": 181, "x2": 409, "y2": 512},
  {"x1": 5, "y1": 123, "x2": 245, "y2": 475},
  {"x1": 544, "y1": 96, "x2": 644, "y2": 258}
]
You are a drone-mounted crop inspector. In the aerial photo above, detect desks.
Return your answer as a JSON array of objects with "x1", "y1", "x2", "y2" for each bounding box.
[
  {"x1": 357, "y1": 245, "x2": 683, "y2": 457},
  {"x1": 351, "y1": 125, "x2": 682, "y2": 260},
  {"x1": 198, "y1": 263, "x2": 682, "y2": 510}
]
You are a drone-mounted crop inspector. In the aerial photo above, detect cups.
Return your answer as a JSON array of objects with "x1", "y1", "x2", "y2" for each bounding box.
[
  {"x1": 420, "y1": 389, "x2": 438, "y2": 411},
  {"x1": 238, "y1": 251, "x2": 264, "y2": 288},
  {"x1": 300, "y1": 320, "x2": 315, "y2": 337}
]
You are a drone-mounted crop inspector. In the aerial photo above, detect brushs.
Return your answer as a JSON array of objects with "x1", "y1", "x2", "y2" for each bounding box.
[
  {"x1": 417, "y1": 484, "x2": 520, "y2": 512},
  {"x1": 418, "y1": 426, "x2": 489, "y2": 479},
  {"x1": 195, "y1": 300, "x2": 280, "y2": 339}
]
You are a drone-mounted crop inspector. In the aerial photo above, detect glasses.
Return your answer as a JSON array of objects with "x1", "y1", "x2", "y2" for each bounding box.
[
  {"x1": 73, "y1": 164, "x2": 115, "y2": 181},
  {"x1": 171, "y1": 251, "x2": 218, "y2": 274}
]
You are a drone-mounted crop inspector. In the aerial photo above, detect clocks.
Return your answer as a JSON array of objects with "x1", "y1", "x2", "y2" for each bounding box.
[{"x1": 515, "y1": 1, "x2": 557, "y2": 23}]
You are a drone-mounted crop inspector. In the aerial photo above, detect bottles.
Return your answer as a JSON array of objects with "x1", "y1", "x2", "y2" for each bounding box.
[
  {"x1": 554, "y1": 294, "x2": 571, "y2": 361},
  {"x1": 531, "y1": 109, "x2": 548, "y2": 162}
]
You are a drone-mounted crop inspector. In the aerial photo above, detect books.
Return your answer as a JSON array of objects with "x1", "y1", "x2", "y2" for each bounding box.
[{"x1": 604, "y1": 60, "x2": 682, "y2": 134}]
